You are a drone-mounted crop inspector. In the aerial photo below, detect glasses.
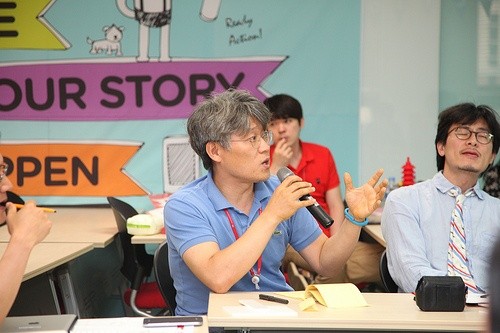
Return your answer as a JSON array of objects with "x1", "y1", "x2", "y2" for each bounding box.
[
  {"x1": 0, "y1": 162, "x2": 9, "y2": 182},
  {"x1": 446, "y1": 127, "x2": 494, "y2": 145},
  {"x1": 217, "y1": 130, "x2": 272, "y2": 147}
]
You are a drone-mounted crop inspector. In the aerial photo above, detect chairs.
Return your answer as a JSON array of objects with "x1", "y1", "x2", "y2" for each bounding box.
[{"x1": 105, "y1": 194, "x2": 169, "y2": 317}]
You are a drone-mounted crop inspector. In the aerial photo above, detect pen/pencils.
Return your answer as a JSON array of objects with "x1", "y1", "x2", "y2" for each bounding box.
[
  {"x1": 259, "y1": 294, "x2": 289, "y2": 304},
  {"x1": 0, "y1": 201, "x2": 55, "y2": 214}
]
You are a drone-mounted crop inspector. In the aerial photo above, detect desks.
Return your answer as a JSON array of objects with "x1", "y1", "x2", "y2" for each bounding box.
[{"x1": 1, "y1": 205, "x2": 492, "y2": 333}]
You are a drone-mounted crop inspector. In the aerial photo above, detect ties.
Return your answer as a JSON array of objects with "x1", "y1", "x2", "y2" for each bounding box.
[{"x1": 446, "y1": 187, "x2": 483, "y2": 294}]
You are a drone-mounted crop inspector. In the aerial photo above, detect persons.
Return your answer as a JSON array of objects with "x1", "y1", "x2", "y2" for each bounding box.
[
  {"x1": 0, "y1": 153, "x2": 51, "y2": 332},
  {"x1": 163, "y1": 86, "x2": 390, "y2": 311},
  {"x1": 380, "y1": 101, "x2": 500, "y2": 301},
  {"x1": 263, "y1": 95, "x2": 385, "y2": 284}
]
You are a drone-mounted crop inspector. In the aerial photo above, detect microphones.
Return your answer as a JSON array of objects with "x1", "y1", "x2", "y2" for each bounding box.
[{"x1": 277, "y1": 167, "x2": 334, "y2": 228}]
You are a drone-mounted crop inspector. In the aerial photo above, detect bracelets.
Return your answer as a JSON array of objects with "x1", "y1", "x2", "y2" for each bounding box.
[{"x1": 344, "y1": 206, "x2": 369, "y2": 227}]
